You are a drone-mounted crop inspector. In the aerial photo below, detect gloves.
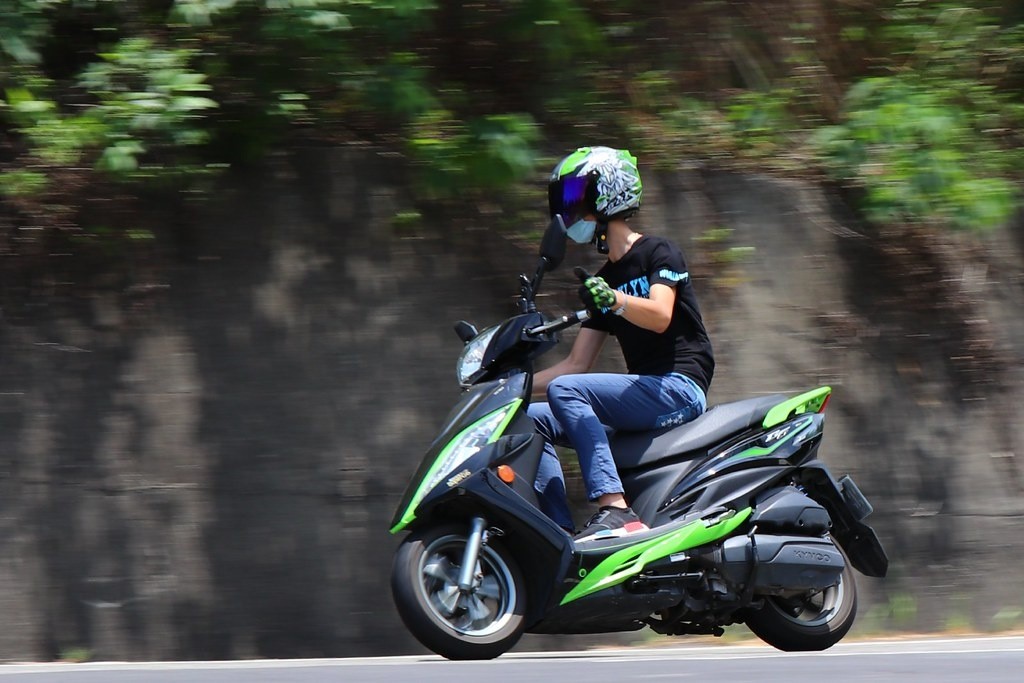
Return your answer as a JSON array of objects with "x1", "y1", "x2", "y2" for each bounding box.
[{"x1": 574, "y1": 266, "x2": 617, "y2": 313}]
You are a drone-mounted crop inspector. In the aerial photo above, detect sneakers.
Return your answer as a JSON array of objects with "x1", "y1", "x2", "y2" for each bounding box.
[{"x1": 573, "y1": 505, "x2": 649, "y2": 544}]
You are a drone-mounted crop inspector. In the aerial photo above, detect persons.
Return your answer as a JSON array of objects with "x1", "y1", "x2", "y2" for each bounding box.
[{"x1": 523, "y1": 145, "x2": 714, "y2": 543}]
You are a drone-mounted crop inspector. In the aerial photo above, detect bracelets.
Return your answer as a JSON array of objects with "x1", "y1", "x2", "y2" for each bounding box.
[{"x1": 614, "y1": 290, "x2": 629, "y2": 316}]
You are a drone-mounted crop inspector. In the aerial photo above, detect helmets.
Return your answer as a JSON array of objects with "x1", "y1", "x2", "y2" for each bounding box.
[{"x1": 548, "y1": 146, "x2": 642, "y2": 233}]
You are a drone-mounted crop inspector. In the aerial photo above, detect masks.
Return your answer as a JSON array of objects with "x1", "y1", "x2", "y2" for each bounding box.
[{"x1": 567, "y1": 220, "x2": 597, "y2": 243}]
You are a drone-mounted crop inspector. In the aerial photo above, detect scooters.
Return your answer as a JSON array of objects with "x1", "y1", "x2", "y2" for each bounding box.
[{"x1": 389, "y1": 212, "x2": 891, "y2": 666}]
